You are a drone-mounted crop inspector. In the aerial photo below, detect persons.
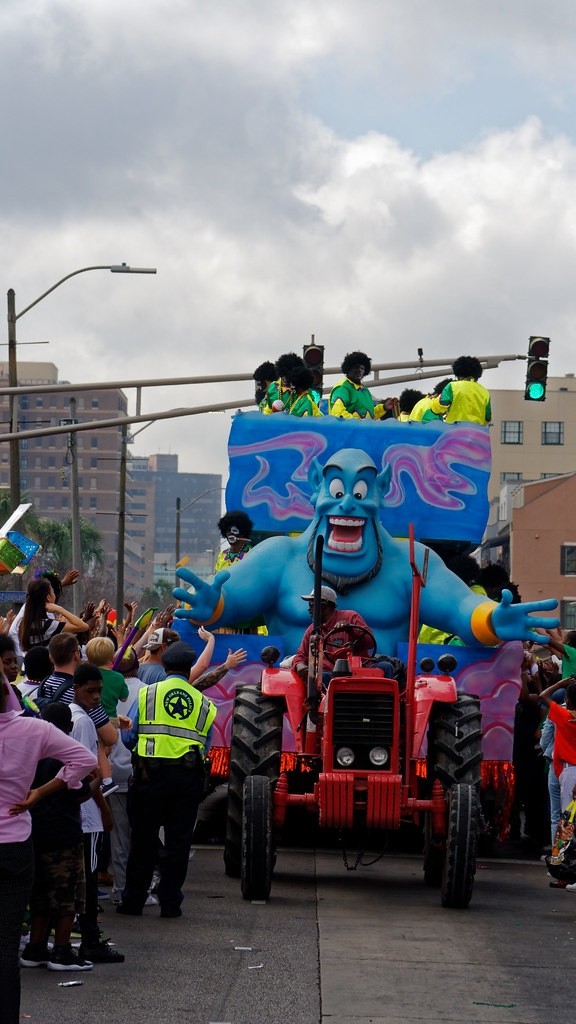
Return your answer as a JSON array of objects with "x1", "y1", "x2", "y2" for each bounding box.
[
  {"x1": 214, "y1": 510, "x2": 254, "y2": 634},
  {"x1": 292, "y1": 586, "x2": 394, "y2": 691},
  {"x1": 0, "y1": 567, "x2": 248, "y2": 1024},
  {"x1": 417, "y1": 555, "x2": 522, "y2": 647},
  {"x1": 252, "y1": 343, "x2": 491, "y2": 425},
  {"x1": 514, "y1": 627, "x2": 576, "y2": 890}
]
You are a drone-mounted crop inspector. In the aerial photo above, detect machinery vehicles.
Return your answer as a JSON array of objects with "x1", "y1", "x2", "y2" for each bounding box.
[{"x1": 220, "y1": 520, "x2": 504, "y2": 906}]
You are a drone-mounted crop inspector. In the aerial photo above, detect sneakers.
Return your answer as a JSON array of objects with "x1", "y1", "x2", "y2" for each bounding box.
[
  {"x1": 19, "y1": 943, "x2": 52, "y2": 966},
  {"x1": 47, "y1": 946, "x2": 93, "y2": 970},
  {"x1": 79, "y1": 938, "x2": 125, "y2": 963},
  {"x1": 20, "y1": 931, "x2": 54, "y2": 950},
  {"x1": 99, "y1": 781, "x2": 119, "y2": 798}
]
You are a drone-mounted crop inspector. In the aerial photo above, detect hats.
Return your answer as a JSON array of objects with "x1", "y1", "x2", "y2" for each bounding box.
[
  {"x1": 162, "y1": 641, "x2": 196, "y2": 666},
  {"x1": 551, "y1": 688, "x2": 565, "y2": 700},
  {"x1": 142, "y1": 628, "x2": 180, "y2": 651},
  {"x1": 301, "y1": 586, "x2": 336, "y2": 603}
]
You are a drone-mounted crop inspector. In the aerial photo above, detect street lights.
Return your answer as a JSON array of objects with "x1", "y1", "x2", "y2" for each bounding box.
[
  {"x1": 5, "y1": 260, "x2": 158, "y2": 611},
  {"x1": 117, "y1": 401, "x2": 230, "y2": 638}
]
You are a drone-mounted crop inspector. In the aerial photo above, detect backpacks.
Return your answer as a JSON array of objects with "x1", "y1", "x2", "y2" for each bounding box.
[{"x1": 24, "y1": 675, "x2": 74, "y2": 718}]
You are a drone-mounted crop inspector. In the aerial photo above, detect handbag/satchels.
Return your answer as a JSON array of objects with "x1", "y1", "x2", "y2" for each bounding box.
[{"x1": 545, "y1": 799, "x2": 576, "y2": 880}]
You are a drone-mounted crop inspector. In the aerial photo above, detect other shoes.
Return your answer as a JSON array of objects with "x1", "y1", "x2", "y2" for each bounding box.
[
  {"x1": 145, "y1": 895, "x2": 158, "y2": 905},
  {"x1": 549, "y1": 880, "x2": 566, "y2": 888},
  {"x1": 98, "y1": 890, "x2": 109, "y2": 900},
  {"x1": 70, "y1": 920, "x2": 104, "y2": 937},
  {"x1": 116, "y1": 904, "x2": 143, "y2": 915},
  {"x1": 160, "y1": 905, "x2": 182, "y2": 918},
  {"x1": 566, "y1": 882, "x2": 576, "y2": 893},
  {"x1": 98, "y1": 878, "x2": 114, "y2": 885}
]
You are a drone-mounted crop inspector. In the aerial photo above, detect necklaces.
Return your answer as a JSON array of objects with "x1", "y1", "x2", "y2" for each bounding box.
[
  {"x1": 280, "y1": 385, "x2": 291, "y2": 407},
  {"x1": 228, "y1": 544, "x2": 244, "y2": 566}
]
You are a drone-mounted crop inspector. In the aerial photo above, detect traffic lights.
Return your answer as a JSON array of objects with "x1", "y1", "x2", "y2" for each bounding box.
[
  {"x1": 304, "y1": 344, "x2": 325, "y2": 398},
  {"x1": 522, "y1": 335, "x2": 550, "y2": 402}
]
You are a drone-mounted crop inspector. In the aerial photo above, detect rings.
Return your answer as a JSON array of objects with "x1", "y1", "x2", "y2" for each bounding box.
[{"x1": 238, "y1": 658, "x2": 240, "y2": 660}]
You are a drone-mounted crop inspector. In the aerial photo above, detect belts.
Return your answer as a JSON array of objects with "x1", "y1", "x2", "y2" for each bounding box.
[{"x1": 562, "y1": 761, "x2": 575, "y2": 768}]
